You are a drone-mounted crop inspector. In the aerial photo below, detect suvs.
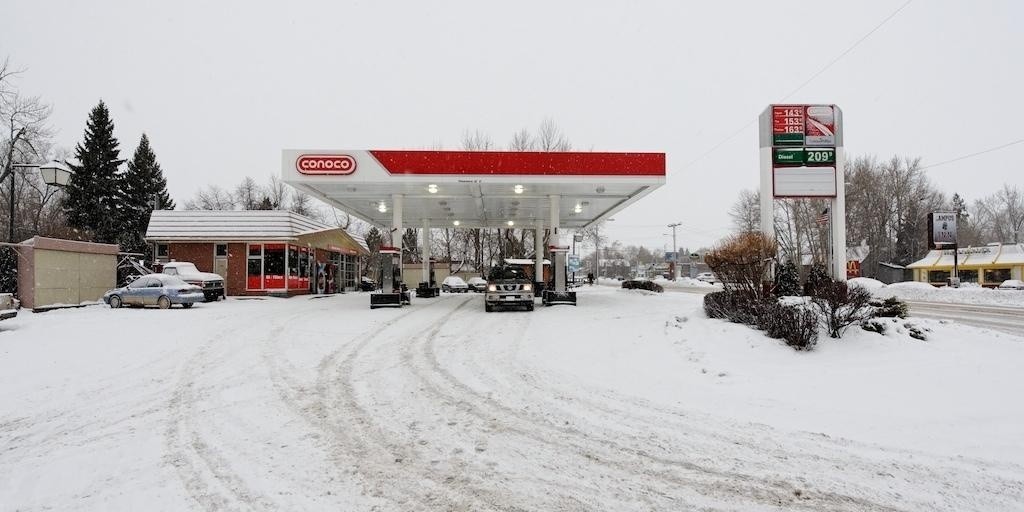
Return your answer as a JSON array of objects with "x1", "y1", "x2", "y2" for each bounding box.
[{"x1": 485, "y1": 267, "x2": 534, "y2": 312}]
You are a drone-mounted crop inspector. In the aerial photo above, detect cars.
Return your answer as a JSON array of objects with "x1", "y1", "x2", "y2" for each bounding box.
[
  {"x1": 1000, "y1": 278, "x2": 1024, "y2": 290},
  {"x1": 442, "y1": 275, "x2": 468, "y2": 292},
  {"x1": 468, "y1": 276, "x2": 486, "y2": 292},
  {"x1": 697, "y1": 271, "x2": 716, "y2": 284},
  {"x1": 102, "y1": 274, "x2": 204, "y2": 309}
]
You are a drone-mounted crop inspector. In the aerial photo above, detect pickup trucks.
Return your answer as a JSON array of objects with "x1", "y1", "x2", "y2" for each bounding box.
[{"x1": 126, "y1": 258, "x2": 224, "y2": 302}]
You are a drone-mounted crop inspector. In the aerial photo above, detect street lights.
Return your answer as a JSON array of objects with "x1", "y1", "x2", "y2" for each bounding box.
[
  {"x1": 8, "y1": 156, "x2": 71, "y2": 243},
  {"x1": 596, "y1": 217, "x2": 616, "y2": 282},
  {"x1": 668, "y1": 221, "x2": 682, "y2": 282}
]
